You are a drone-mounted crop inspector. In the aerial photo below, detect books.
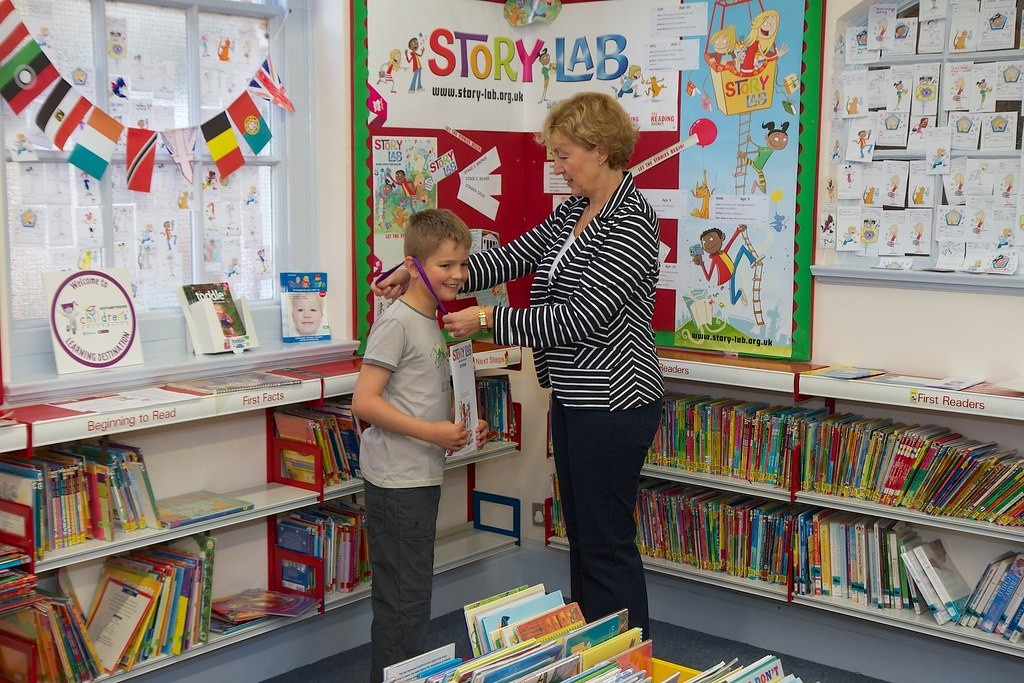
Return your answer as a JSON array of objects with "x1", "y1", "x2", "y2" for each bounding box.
[{"x1": 0, "y1": 272, "x2": 1024, "y2": 683}]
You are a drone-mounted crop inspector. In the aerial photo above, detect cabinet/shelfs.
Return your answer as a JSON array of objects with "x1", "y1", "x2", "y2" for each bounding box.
[
  {"x1": 547, "y1": 347, "x2": 1024, "y2": 658},
  {"x1": 0, "y1": 341, "x2": 522, "y2": 683}
]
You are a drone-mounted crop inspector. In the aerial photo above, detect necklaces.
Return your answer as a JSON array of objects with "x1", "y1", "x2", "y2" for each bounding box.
[{"x1": 576, "y1": 204, "x2": 603, "y2": 238}]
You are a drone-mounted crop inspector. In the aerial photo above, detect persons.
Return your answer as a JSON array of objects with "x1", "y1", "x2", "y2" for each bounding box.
[
  {"x1": 370, "y1": 92, "x2": 666, "y2": 649},
  {"x1": 1011, "y1": 559, "x2": 1024, "y2": 577},
  {"x1": 353, "y1": 209, "x2": 488, "y2": 683},
  {"x1": 929, "y1": 540, "x2": 954, "y2": 574},
  {"x1": 214, "y1": 303, "x2": 235, "y2": 336},
  {"x1": 291, "y1": 293, "x2": 324, "y2": 336}
]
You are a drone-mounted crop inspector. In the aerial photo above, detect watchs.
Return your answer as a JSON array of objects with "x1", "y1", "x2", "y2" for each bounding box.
[{"x1": 479, "y1": 304, "x2": 492, "y2": 335}]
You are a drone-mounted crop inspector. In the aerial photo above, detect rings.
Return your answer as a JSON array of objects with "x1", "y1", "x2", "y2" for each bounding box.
[{"x1": 449, "y1": 331, "x2": 457, "y2": 339}]
